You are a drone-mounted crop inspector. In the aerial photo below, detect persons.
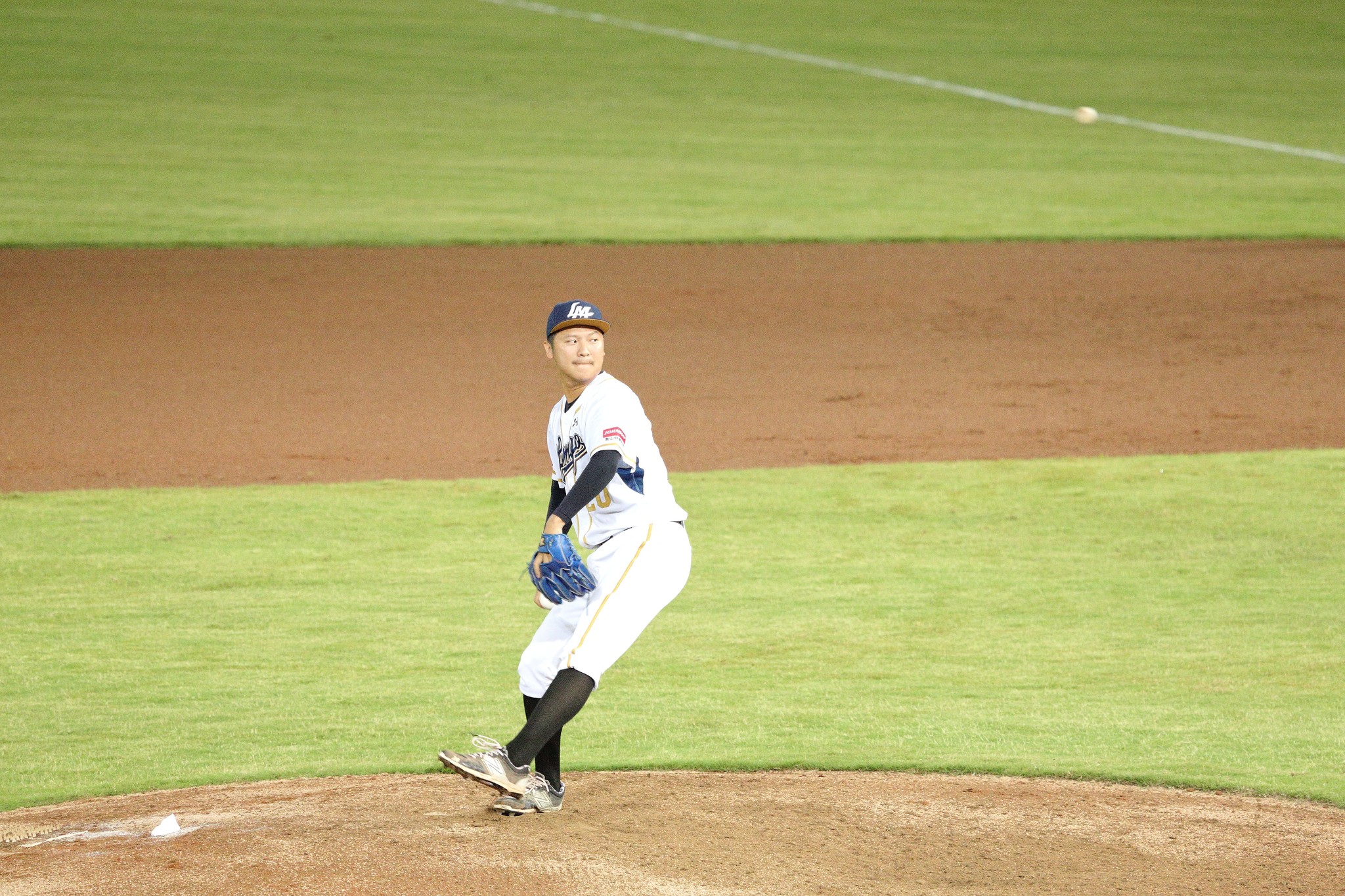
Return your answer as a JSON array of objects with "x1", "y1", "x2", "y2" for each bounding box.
[{"x1": 437, "y1": 301, "x2": 693, "y2": 813}]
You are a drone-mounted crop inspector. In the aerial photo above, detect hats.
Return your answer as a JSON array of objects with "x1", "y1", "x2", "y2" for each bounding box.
[{"x1": 546, "y1": 300, "x2": 610, "y2": 340}]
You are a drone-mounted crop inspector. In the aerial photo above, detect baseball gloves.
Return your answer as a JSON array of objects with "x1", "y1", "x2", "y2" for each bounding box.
[{"x1": 526, "y1": 532, "x2": 597, "y2": 604}]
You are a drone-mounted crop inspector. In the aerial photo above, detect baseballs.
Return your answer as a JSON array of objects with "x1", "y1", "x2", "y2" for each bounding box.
[
  {"x1": 1076, "y1": 106, "x2": 1098, "y2": 124},
  {"x1": 539, "y1": 589, "x2": 561, "y2": 610}
]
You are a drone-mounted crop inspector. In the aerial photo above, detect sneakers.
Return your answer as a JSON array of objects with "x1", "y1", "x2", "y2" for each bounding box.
[
  {"x1": 437, "y1": 733, "x2": 531, "y2": 799},
  {"x1": 493, "y1": 771, "x2": 565, "y2": 817}
]
